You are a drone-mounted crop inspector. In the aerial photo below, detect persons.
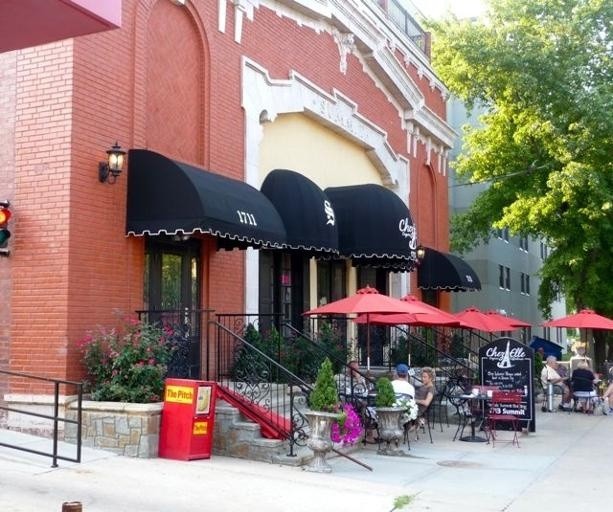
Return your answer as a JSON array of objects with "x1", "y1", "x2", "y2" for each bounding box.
[
  {"x1": 571, "y1": 361, "x2": 595, "y2": 414},
  {"x1": 333, "y1": 359, "x2": 379, "y2": 444},
  {"x1": 536, "y1": 347, "x2": 544, "y2": 364},
  {"x1": 388, "y1": 363, "x2": 416, "y2": 422},
  {"x1": 595, "y1": 360, "x2": 612, "y2": 417},
  {"x1": 540, "y1": 355, "x2": 572, "y2": 412},
  {"x1": 415, "y1": 366, "x2": 435, "y2": 415}
]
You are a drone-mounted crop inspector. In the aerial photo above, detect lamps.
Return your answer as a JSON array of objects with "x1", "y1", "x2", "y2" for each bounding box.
[
  {"x1": 97, "y1": 139, "x2": 127, "y2": 187},
  {"x1": 415, "y1": 240, "x2": 425, "y2": 265}
]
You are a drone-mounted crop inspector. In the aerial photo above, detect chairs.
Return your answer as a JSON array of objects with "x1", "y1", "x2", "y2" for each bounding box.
[{"x1": 329, "y1": 345, "x2": 612, "y2": 449}]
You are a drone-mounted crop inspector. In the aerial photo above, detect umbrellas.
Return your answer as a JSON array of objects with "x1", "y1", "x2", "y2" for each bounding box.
[
  {"x1": 451, "y1": 305, "x2": 514, "y2": 332},
  {"x1": 302, "y1": 283, "x2": 428, "y2": 373},
  {"x1": 481, "y1": 308, "x2": 531, "y2": 343},
  {"x1": 215, "y1": 383, "x2": 373, "y2": 473},
  {"x1": 351, "y1": 293, "x2": 459, "y2": 367},
  {"x1": 536, "y1": 307, "x2": 613, "y2": 363}
]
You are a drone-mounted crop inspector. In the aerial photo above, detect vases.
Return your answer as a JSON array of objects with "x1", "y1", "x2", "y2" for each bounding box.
[{"x1": 303, "y1": 407, "x2": 339, "y2": 474}]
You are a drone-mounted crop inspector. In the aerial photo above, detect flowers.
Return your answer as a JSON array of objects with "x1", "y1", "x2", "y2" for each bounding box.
[{"x1": 305, "y1": 356, "x2": 363, "y2": 446}]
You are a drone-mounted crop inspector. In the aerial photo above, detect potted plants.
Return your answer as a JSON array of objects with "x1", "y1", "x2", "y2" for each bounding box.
[{"x1": 370, "y1": 376, "x2": 408, "y2": 454}]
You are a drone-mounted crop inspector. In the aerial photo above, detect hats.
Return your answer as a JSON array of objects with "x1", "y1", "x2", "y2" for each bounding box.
[{"x1": 396, "y1": 365, "x2": 409, "y2": 374}]
